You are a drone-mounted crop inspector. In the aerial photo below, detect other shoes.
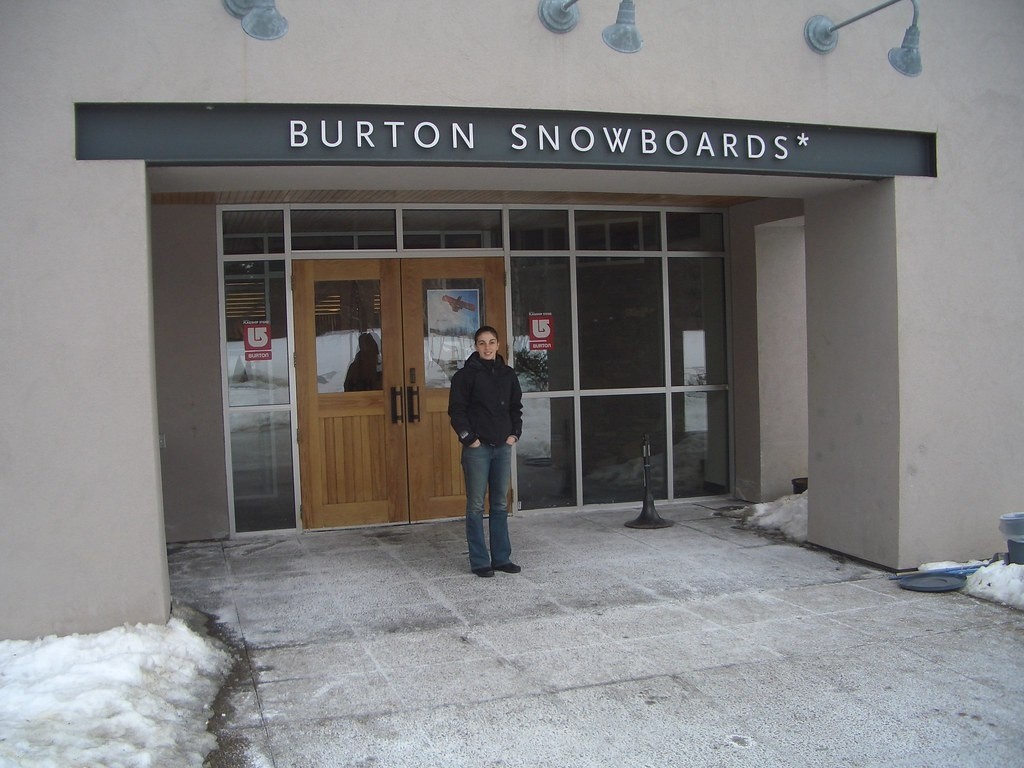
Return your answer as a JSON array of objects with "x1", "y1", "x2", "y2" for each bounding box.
[
  {"x1": 472, "y1": 567, "x2": 494, "y2": 577},
  {"x1": 494, "y1": 563, "x2": 521, "y2": 573}
]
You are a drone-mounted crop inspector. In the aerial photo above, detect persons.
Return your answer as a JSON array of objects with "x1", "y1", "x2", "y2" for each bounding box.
[
  {"x1": 343, "y1": 333, "x2": 380, "y2": 392},
  {"x1": 447, "y1": 325, "x2": 525, "y2": 578}
]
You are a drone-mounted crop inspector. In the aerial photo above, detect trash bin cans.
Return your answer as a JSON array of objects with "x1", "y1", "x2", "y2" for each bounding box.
[{"x1": 1000, "y1": 512, "x2": 1024, "y2": 564}]
